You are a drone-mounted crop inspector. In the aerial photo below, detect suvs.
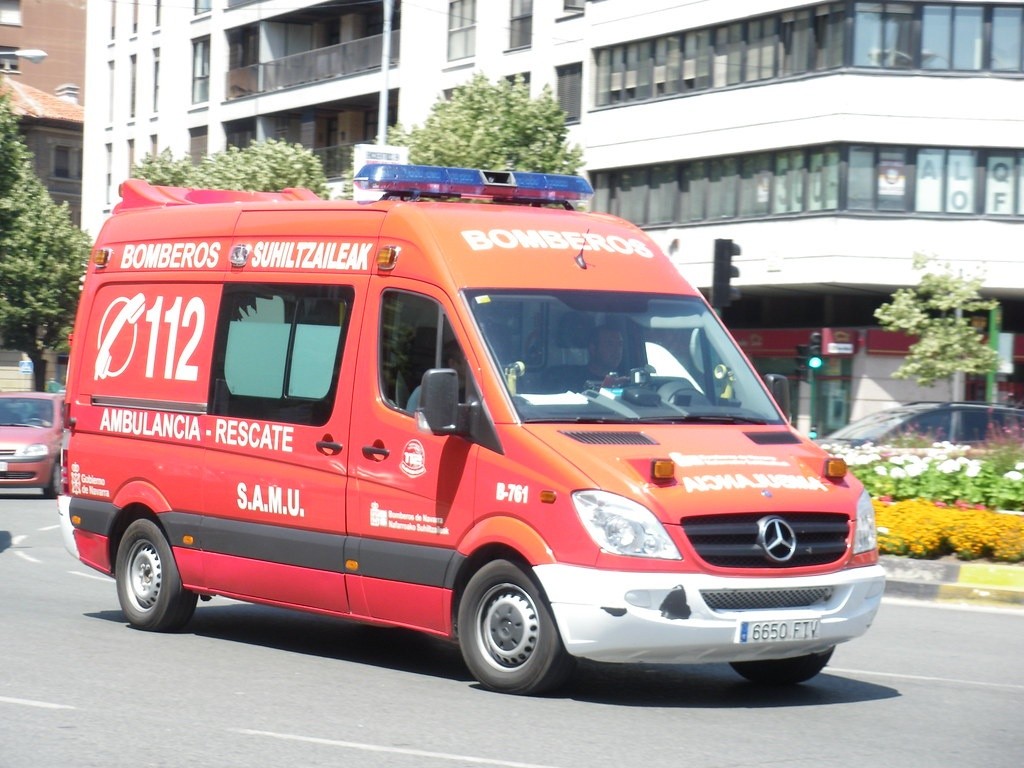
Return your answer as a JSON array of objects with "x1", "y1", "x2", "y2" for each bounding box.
[{"x1": 812, "y1": 398, "x2": 1024, "y2": 452}]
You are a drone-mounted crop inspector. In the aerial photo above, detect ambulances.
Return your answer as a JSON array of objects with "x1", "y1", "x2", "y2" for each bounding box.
[{"x1": 63, "y1": 163, "x2": 885, "y2": 697}]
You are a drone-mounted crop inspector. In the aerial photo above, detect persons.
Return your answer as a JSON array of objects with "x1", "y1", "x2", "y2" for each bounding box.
[
  {"x1": 559, "y1": 323, "x2": 630, "y2": 393},
  {"x1": 29, "y1": 403, "x2": 52, "y2": 426},
  {"x1": 406, "y1": 339, "x2": 467, "y2": 412}
]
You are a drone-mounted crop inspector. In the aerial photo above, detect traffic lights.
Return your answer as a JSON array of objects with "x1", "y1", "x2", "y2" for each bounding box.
[
  {"x1": 795, "y1": 343, "x2": 809, "y2": 381},
  {"x1": 710, "y1": 236, "x2": 740, "y2": 308},
  {"x1": 808, "y1": 331, "x2": 823, "y2": 372}
]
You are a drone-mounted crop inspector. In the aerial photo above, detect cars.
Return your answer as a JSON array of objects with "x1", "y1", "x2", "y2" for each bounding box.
[{"x1": 1, "y1": 390, "x2": 66, "y2": 500}]
